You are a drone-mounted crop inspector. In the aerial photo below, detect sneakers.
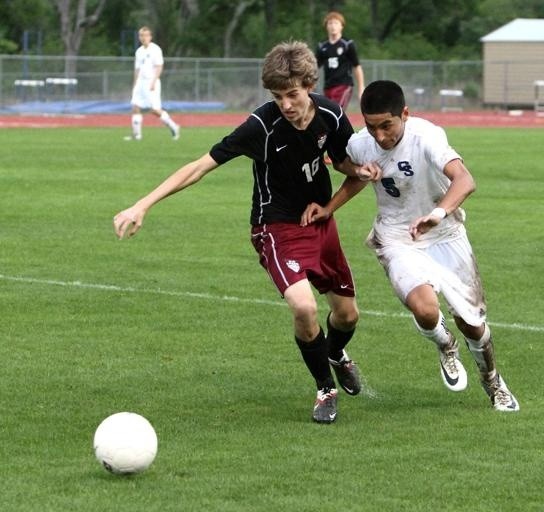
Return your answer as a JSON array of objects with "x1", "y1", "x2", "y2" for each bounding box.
[
  {"x1": 123, "y1": 133, "x2": 142, "y2": 141},
  {"x1": 170, "y1": 125, "x2": 180, "y2": 140},
  {"x1": 478, "y1": 372, "x2": 520, "y2": 412},
  {"x1": 313, "y1": 377, "x2": 338, "y2": 424},
  {"x1": 439, "y1": 339, "x2": 468, "y2": 391},
  {"x1": 324, "y1": 335, "x2": 361, "y2": 395}
]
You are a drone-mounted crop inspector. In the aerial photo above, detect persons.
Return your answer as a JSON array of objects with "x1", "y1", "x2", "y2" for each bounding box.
[
  {"x1": 112, "y1": 33, "x2": 372, "y2": 424},
  {"x1": 122, "y1": 24, "x2": 180, "y2": 142},
  {"x1": 313, "y1": 9, "x2": 366, "y2": 165},
  {"x1": 300, "y1": 80, "x2": 520, "y2": 416}
]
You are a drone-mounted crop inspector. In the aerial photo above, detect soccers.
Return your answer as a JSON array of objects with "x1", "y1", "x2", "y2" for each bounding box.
[{"x1": 94, "y1": 411, "x2": 158, "y2": 475}]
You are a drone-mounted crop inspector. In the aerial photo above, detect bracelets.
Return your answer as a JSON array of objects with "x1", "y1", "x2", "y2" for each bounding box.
[{"x1": 430, "y1": 206, "x2": 449, "y2": 222}]
